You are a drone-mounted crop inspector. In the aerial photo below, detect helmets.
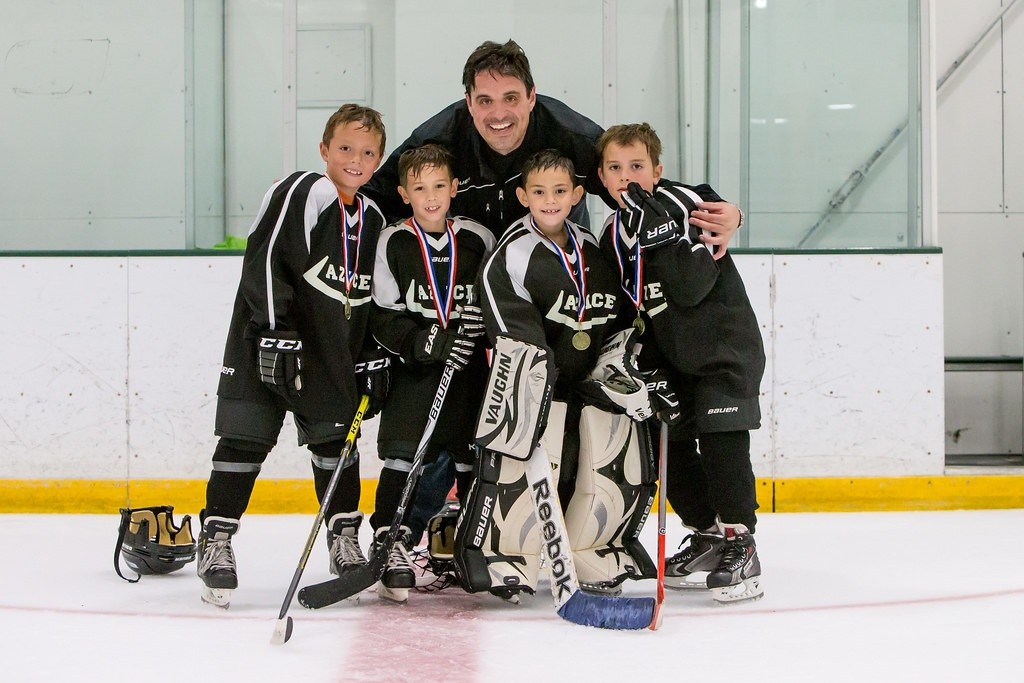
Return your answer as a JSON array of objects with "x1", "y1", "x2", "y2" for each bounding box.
[
  {"x1": 114, "y1": 506, "x2": 199, "y2": 583},
  {"x1": 428, "y1": 501, "x2": 463, "y2": 564}
]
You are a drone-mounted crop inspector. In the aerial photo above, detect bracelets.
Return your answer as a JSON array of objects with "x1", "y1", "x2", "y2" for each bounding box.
[{"x1": 736, "y1": 207, "x2": 745, "y2": 228}]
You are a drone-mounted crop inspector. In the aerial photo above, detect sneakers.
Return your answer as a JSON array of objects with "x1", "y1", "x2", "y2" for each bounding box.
[
  {"x1": 326, "y1": 511, "x2": 368, "y2": 606},
  {"x1": 707, "y1": 517, "x2": 765, "y2": 602},
  {"x1": 369, "y1": 524, "x2": 415, "y2": 601},
  {"x1": 664, "y1": 521, "x2": 725, "y2": 589},
  {"x1": 196, "y1": 509, "x2": 240, "y2": 609}
]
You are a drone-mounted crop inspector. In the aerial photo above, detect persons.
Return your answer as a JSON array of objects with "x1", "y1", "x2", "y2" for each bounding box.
[
  {"x1": 473, "y1": 148, "x2": 654, "y2": 605},
  {"x1": 197, "y1": 103, "x2": 386, "y2": 608},
  {"x1": 591, "y1": 124, "x2": 767, "y2": 605},
  {"x1": 369, "y1": 146, "x2": 498, "y2": 605},
  {"x1": 359, "y1": 40, "x2": 737, "y2": 261}
]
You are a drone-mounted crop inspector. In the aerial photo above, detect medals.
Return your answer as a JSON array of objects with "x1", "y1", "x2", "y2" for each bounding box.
[
  {"x1": 345, "y1": 302, "x2": 351, "y2": 320},
  {"x1": 632, "y1": 318, "x2": 645, "y2": 335},
  {"x1": 572, "y1": 332, "x2": 591, "y2": 350}
]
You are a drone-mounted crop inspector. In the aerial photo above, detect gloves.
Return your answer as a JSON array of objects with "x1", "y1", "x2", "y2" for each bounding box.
[
  {"x1": 646, "y1": 372, "x2": 682, "y2": 427},
  {"x1": 622, "y1": 182, "x2": 680, "y2": 256},
  {"x1": 457, "y1": 304, "x2": 486, "y2": 337},
  {"x1": 414, "y1": 324, "x2": 476, "y2": 372},
  {"x1": 353, "y1": 347, "x2": 389, "y2": 420},
  {"x1": 255, "y1": 328, "x2": 302, "y2": 411}
]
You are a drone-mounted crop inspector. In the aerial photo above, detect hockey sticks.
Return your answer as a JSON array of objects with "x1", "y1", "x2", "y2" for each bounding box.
[
  {"x1": 521, "y1": 444, "x2": 657, "y2": 631},
  {"x1": 271, "y1": 392, "x2": 370, "y2": 646},
  {"x1": 296, "y1": 322, "x2": 464, "y2": 609},
  {"x1": 649, "y1": 423, "x2": 669, "y2": 630}
]
